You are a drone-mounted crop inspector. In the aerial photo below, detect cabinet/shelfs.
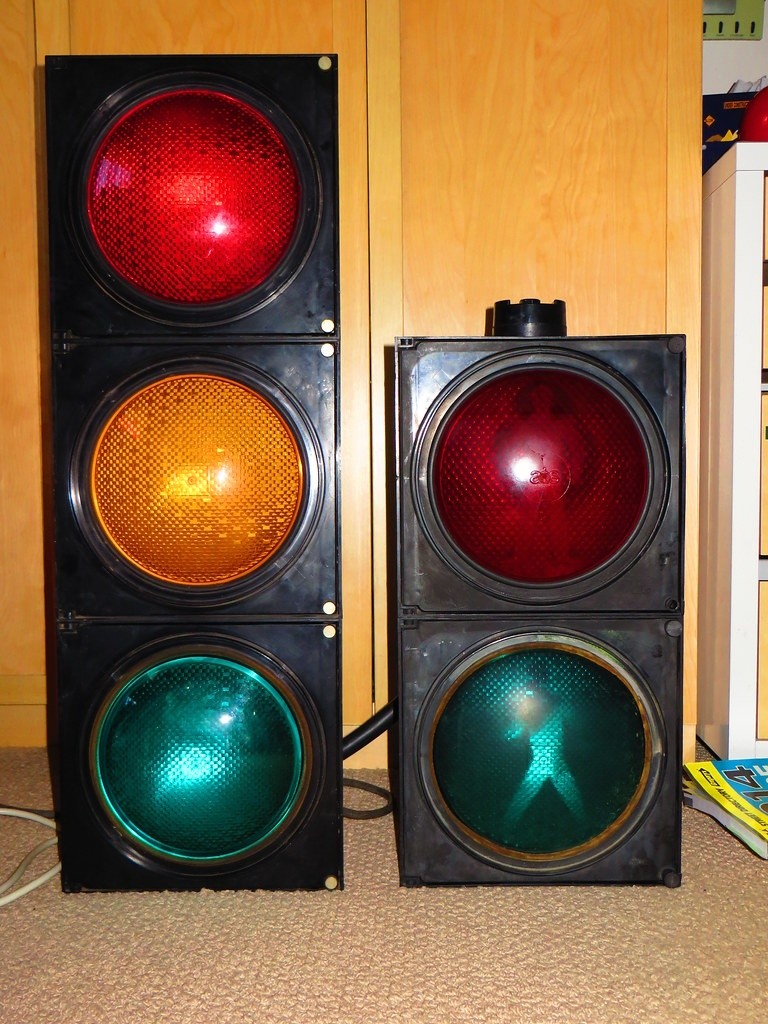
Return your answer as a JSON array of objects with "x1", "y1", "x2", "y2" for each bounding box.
[{"x1": 701, "y1": 140, "x2": 768, "y2": 763}]
